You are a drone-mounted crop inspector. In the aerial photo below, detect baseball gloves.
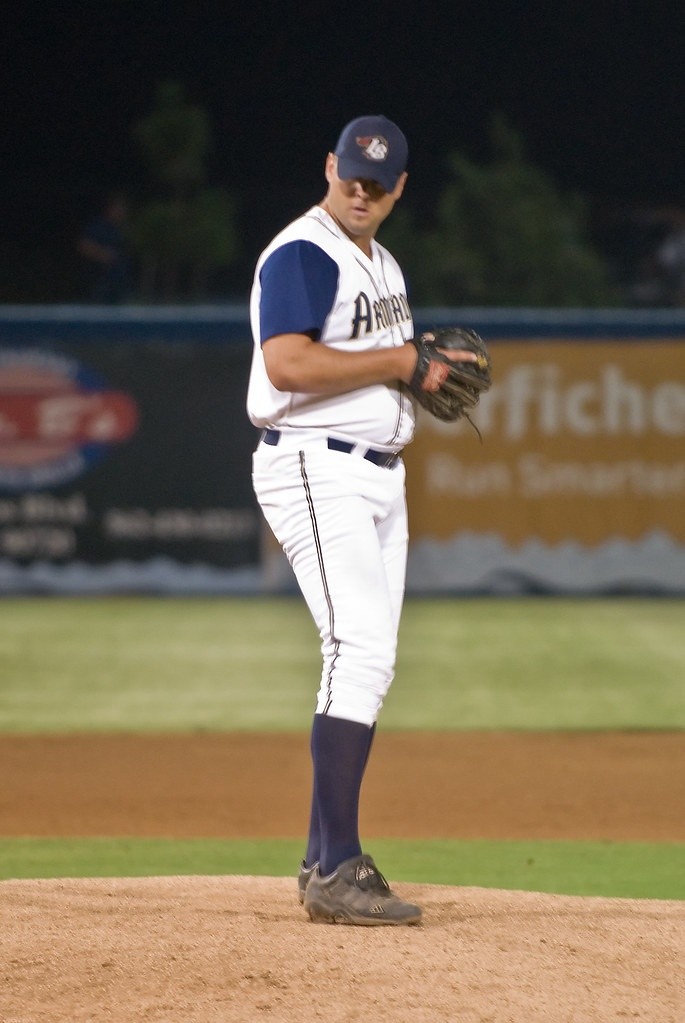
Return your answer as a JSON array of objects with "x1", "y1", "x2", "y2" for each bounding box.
[{"x1": 405, "y1": 328, "x2": 494, "y2": 425}]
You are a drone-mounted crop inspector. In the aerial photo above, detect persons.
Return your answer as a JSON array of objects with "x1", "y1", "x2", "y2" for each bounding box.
[{"x1": 246, "y1": 113, "x2": 492, "y2": 926}]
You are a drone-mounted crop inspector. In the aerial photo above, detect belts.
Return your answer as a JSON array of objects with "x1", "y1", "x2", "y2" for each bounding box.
[{"x1": 263, "y1": 427, "x2": 403, "y2": 469}]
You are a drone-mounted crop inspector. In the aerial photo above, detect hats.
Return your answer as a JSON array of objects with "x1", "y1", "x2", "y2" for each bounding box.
[{"x1": 334, "y1": 113, "x2": 409, "y2": 193}]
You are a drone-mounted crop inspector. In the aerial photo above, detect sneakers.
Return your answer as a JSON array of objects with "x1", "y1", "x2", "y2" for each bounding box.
[
  {"x1": 298, "y1": 860, "x2": 318, "y2": 904},
  {"x1": 304, "y1": 853, "x2": 423, "y2": 928}
]
